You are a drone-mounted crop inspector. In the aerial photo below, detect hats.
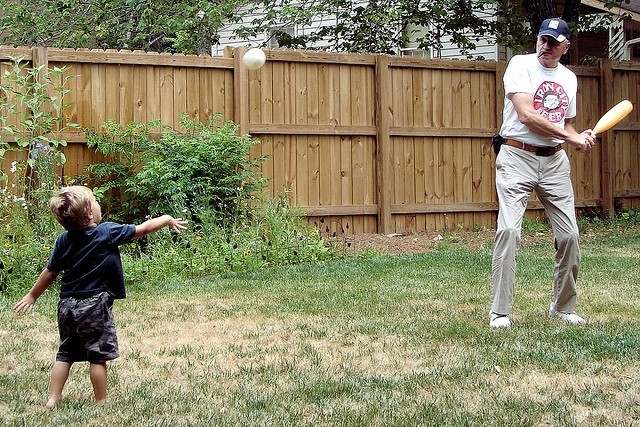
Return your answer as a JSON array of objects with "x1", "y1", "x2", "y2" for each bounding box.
[{"x1": 538, "y1": 17, "x2": 571, "y2": 42}]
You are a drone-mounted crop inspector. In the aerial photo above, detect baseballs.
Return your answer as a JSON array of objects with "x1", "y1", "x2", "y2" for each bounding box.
[{"x1": 242, "y1": 48, "x2": 266, "y2": 71}]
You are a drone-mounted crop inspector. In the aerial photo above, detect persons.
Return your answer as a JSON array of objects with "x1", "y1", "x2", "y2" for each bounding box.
[
  {"x1": 14, "y1": 185, "x2": 188, "y2": 410},
  {"x1": 490, "y1": 18, "x2": 596, "y2": 327}
]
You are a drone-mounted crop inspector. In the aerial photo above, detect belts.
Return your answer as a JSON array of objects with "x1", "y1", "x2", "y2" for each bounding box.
[{"x1": 502, "y1": 139, "x2": 563, "y2": 157}]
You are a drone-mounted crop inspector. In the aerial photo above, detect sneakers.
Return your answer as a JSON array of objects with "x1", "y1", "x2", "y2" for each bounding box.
[
  {"x1": 548, "y1": 302, "x2": 586, "y2": 326},
  {"x1": 489, "y1": 310, "x2": 511, "y2": 329}
]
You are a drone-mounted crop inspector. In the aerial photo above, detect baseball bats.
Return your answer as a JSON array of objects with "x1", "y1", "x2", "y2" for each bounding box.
[{"x1": 575, "y1": 100, "x2": 633, "y2": 151}]
